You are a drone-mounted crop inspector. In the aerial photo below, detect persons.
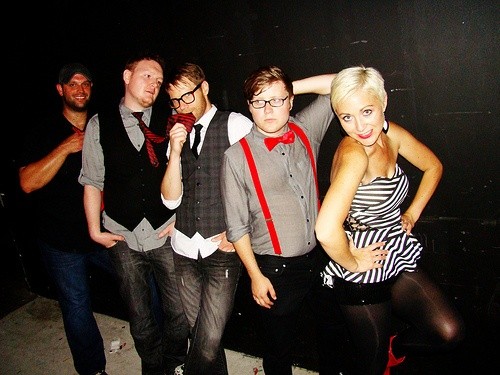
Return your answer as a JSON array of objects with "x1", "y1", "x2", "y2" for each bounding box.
[
  {"x1": 19, "y1": 64, "x2": 130, "y2": 375},
  {"x1": 314, "y1": 64, "x2": 466, "y2": 375},
  {"x1": 160, "y1": 63, "x2": 253, "y2": 375},
  {"x1": 219, "y1": 66, "x2": 339, "y2": 375},
  {"x1": 78, "y1": 54, "x2": 188, "y2": 375}
]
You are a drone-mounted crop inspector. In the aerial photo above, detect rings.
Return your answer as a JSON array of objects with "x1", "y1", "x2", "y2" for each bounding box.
[
  {"x1": 401, "y1": 219, "x2": 404, "y2": 222},
  {"x1": 404, "y1": 222, "x2": 408, "y2": 226}
]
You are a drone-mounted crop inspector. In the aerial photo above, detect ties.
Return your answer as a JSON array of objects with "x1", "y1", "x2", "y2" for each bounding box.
[
  {"x1": 131, "y1": 112, "x2": 196, "y2": 168},
  {"x1": 191, "y1": 124, "x2": 203, "y2": 159}
]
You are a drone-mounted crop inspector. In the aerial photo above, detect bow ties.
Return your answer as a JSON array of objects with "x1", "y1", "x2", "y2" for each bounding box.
[{"x1": 264, "y1": 131, "x2": 295, "y2": 151}]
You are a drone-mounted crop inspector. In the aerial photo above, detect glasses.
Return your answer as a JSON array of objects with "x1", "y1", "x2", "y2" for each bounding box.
[
  {"x1": 168, "y1": 82, "x2": 202, "y2": 109},
  {"x1": 249, "y1": 95, "x2": 290, "y2": 109}
]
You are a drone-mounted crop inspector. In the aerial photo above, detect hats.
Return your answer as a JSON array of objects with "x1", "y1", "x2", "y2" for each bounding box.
[{"x1": 58, "y1": 62, "x2": 92, "y2": 83}]
